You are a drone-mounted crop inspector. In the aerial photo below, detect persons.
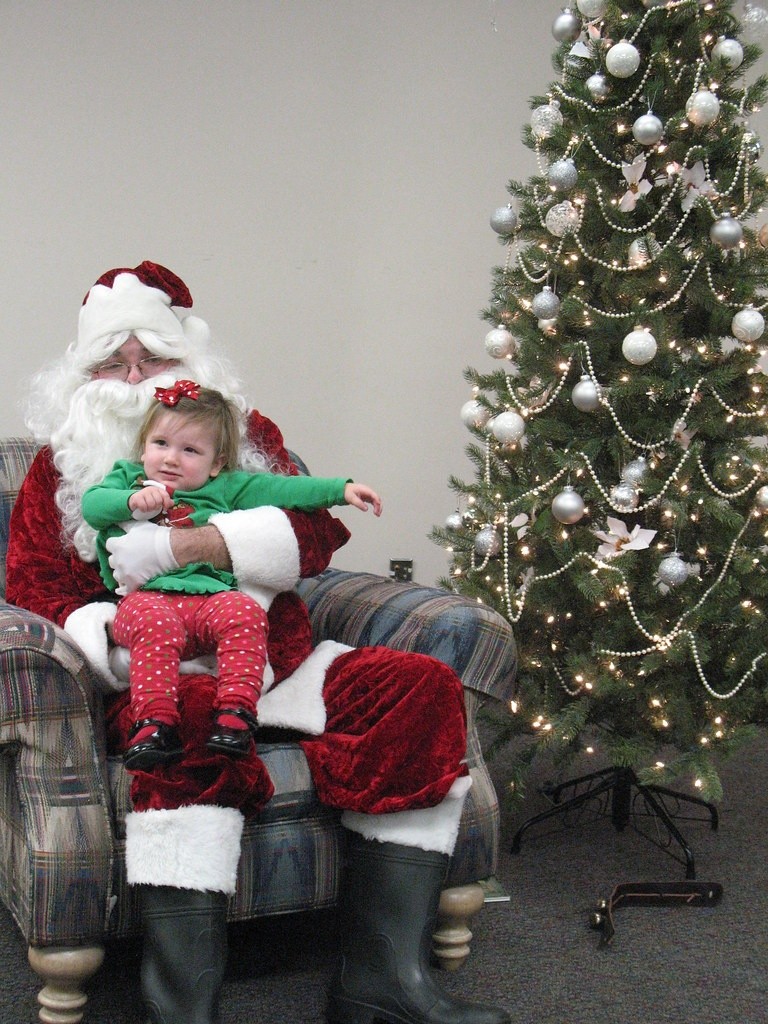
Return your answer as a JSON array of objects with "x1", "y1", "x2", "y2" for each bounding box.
[
  {"x1": 4, "y1": 262, "x2": 513, "y2": 1024},
  {"x1": 81, "y1": 380, "x2": 382, "y2": 768}
]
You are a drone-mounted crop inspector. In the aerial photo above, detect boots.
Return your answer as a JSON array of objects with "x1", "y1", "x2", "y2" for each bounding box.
[
  {"x1": 325, "y1": 798, "x2": 511, "y2": 1024},
  {"x1": 126, "y1": 803, "x2": 245, "y2": 1024}
]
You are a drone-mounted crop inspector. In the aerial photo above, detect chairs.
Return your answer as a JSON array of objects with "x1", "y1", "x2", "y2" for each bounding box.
[{"x1": 0, "y1": 435, "x2": 517, "y2": 1023}]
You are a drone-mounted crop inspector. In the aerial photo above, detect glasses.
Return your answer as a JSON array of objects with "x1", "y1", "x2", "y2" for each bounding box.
[{"x1": 85, "y1": 357, "x2": 168, "y2": 383}]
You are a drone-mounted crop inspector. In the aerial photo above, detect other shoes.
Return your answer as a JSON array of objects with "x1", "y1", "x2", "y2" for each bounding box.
[
  {"x1": 121, "y1": 718, "x2": 187, "y2": 770},
  {"x1": 205, "y1": 708, "x2": 259, "y2": 755}
]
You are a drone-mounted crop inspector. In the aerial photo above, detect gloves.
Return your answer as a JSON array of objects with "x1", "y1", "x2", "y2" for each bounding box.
[
  {"x1": 106, "y1": 520, "x2": 181, "y2": 596},
  {"x1": 109, "y1": 647, "x2": 130, "y2": 682}
]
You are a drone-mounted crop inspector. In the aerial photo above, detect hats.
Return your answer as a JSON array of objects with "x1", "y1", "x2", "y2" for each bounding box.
[{"x1": 77, "y1": 260, "x2": 194, "y2": 344}]
